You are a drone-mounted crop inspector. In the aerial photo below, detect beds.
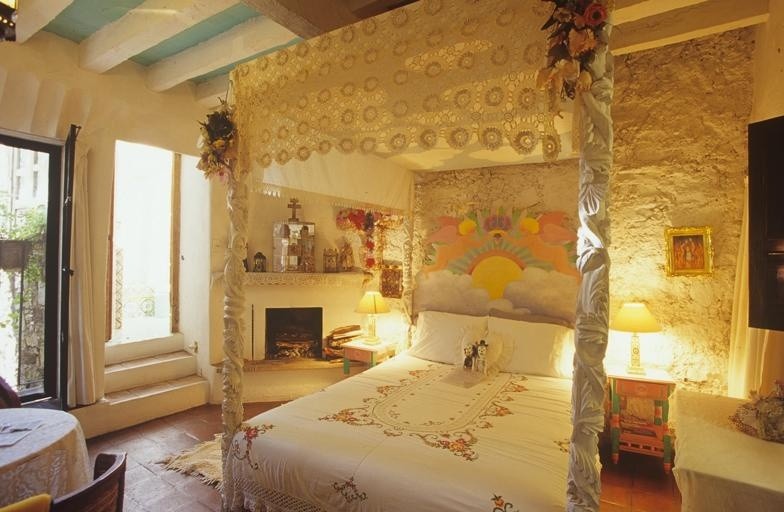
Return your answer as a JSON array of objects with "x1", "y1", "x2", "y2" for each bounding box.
[{"x1": 223, "y1": 1, "x2": 613, "y2": 512}]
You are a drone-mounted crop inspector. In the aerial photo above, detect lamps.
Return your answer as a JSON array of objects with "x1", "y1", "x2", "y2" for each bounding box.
[
  {"x1": 354, "y1": 291, "x2": 391, "y2": 346},
  {"x1": 609, "y1": 302, "x2": 663, "y2": 376},
  {"x1": 0, "y1": 0, "x2": 19, "y2": 42}
]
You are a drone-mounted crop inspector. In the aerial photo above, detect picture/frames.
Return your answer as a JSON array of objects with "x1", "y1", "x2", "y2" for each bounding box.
[{"x1": 663, "y1": 224, "x2": 714, "y2": 278}]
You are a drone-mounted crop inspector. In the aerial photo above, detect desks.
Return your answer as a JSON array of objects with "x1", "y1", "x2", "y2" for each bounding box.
[
  {"x1": 667, "y1": 388, "x2": 784, "y2": 512},
  {"x1": 340, "y1": 341, "x2": 396, "y2": 378}
]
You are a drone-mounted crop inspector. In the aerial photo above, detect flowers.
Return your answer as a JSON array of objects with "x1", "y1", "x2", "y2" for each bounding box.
[
  {"x1": 194, "y1": 100, "x2": 238, "y2": 181},
  {"x1": 539, "y1": 0, "x2": 608, "y2": 104}
]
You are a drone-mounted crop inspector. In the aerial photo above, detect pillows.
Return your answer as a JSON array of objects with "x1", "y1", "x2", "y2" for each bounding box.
[
  {"x1": 406, "y1": 310, "x2": 489, "y2": 365},
  {"x1": 487, "y1": 316, "x2": 575, "y2": 380},
  {"x1": 489, "y1": 308, "x2": 574, "y2": 329}
]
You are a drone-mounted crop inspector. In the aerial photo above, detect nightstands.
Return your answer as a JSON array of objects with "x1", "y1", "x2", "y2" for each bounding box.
[{"x1": 608, "y1": 369, "x2": 676, "y2": 476}]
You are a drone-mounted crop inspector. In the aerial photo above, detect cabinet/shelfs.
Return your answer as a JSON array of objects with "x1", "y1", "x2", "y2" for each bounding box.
[{"x1": 748, "y1": 116, "x2": 784, "y2": 331}]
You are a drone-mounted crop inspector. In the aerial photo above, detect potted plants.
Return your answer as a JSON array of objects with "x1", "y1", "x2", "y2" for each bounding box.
[{"x1": 0, "y1": 203, "x2": 47, "y2": 339}]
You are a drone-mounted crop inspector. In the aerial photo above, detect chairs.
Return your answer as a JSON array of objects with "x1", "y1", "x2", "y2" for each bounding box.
[
  {"x1": 0, "y1": 375, "x2": 21, "y2": 408},
  {"x1": 0, "y1": 494, "x2": 52, "y2": 512},
  {"x1": 52, "y1": 452, "x2": 128, "y2": 512}
]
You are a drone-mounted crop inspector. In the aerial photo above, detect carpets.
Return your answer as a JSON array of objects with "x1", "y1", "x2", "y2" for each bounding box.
[{"x1": 155, "y1": 432, "x2": 225, "y2": 493}]
